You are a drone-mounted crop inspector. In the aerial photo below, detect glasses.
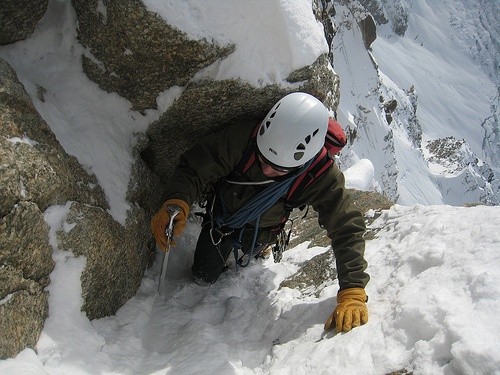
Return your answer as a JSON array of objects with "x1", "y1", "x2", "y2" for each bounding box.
[{"x1": 255, "y1": 146, "x2": 289, "y2": 173}]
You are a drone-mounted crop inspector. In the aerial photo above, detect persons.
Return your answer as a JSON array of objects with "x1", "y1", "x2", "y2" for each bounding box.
[{"x1": 150, "y1": 91, "x2": 370, "y2": 333}]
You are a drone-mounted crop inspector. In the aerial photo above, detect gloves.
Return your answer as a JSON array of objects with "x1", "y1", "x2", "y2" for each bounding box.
[
  {"x1": 150, "y1": 199, "x2": 189, "y2": 250},
  {"x1": 324, "y1": 287, "x2": 368, "y2": 333}
]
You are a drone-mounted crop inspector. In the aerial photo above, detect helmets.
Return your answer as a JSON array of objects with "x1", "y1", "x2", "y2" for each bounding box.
[{"x1": 255, "y1": 92, "x2": 329, "y2": 169}]
